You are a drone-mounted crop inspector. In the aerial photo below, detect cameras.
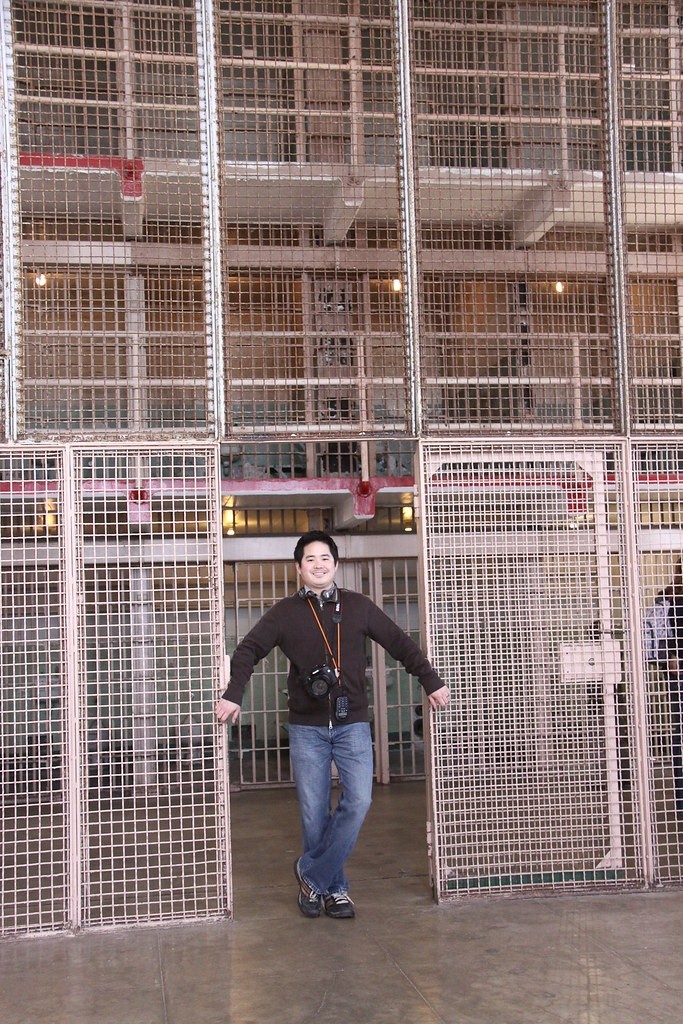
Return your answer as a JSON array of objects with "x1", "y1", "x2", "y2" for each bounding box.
[{"x1": 304, "y1": 665, "x2": 338, "y2": 698}]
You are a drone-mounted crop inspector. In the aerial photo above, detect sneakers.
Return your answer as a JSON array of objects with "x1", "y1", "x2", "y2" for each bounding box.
[
  {"x1": 324, "y1": 891, "x2": 354, "y2": 918},
  {"x1": 294, "y1": 857, "x2": 321, "y2": 917}
]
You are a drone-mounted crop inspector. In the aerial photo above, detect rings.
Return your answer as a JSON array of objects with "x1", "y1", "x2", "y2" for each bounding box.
[{"x1": 446, "y1": 694, "x2": 449, "y2": 698}]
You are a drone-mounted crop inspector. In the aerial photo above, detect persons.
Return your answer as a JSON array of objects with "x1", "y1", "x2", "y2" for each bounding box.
[
  {"x1": 215, "y1": 531, "x2": 451, "y2": 918},
  {"x1": 655, "y1": 557, "x2": 683, "y2": 833}
]
